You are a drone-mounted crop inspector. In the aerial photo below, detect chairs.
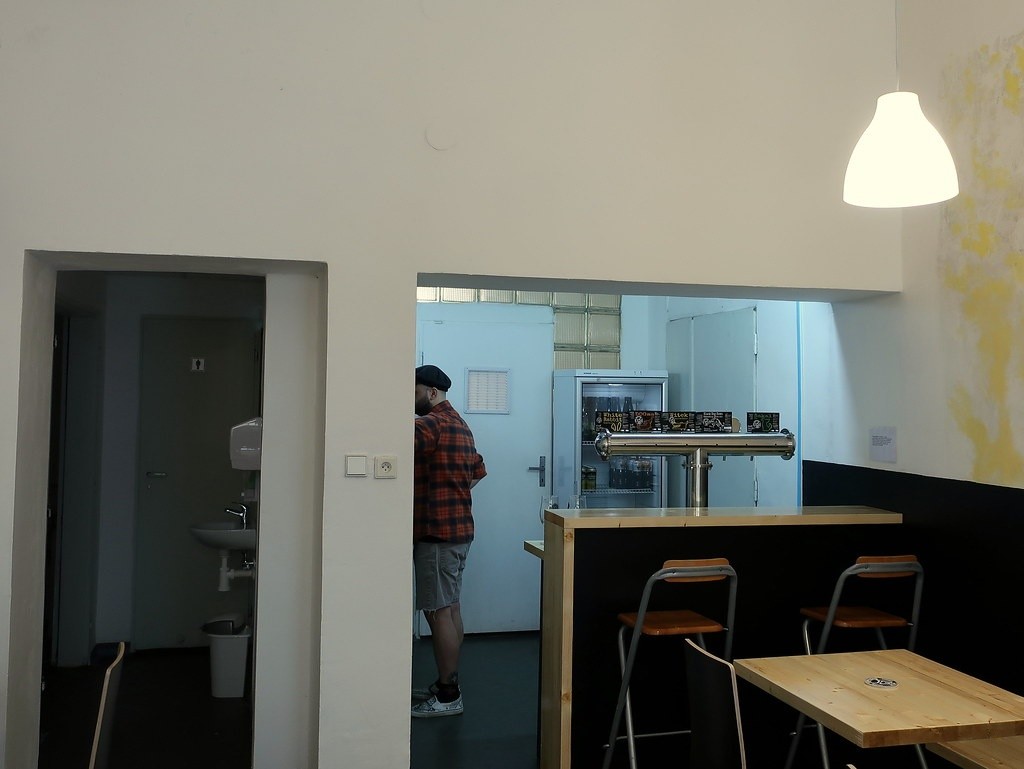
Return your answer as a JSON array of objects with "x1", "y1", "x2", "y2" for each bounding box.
[
  {"x1": 89, "y1": 641, "x2": 125, "y2": 769},
  {"x1": 684, "y1": 638, "x2": 747, "y2": 769}
]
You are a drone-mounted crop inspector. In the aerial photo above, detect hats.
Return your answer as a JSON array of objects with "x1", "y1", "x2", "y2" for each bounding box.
[{"x1": 415, "y1": 365, "x2": 452, "y2": 392}]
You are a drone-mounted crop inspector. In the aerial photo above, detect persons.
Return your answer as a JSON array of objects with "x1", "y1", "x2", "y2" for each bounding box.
[{"x1": 410, "y1": 365, "x2": 488, "y2": 718}]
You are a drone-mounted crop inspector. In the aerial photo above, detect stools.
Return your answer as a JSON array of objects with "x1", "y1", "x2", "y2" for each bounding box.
[
  {"x1": 785, "y1": 555, "x2": 929, "y2": 769},
  {"x1": 926, "y1": 733, "x2": 1024, "y2": 769},
  {"x1": 601, "y1": 558, "x2": 738, "y2": 769}
]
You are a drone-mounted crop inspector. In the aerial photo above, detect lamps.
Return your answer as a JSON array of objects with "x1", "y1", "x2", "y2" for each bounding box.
[{"x1": 843, "y1": 0, "x2": 960, "y2": 208}]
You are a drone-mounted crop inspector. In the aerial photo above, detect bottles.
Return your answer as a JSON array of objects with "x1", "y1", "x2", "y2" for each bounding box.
[
  {"x1": 583, "y1": 396, "x2": 634, "y2": 441},
  {"x1": 582, "y1": 464, "x2": 596, "y2": 490},
  {"x1": 609, "y1": 455, "x2": 653, "y2": 491}
]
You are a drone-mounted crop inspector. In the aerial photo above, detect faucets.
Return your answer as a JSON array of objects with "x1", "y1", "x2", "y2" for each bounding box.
[{"x1": 224, "y1": 501, "x2": 249, "y2": 529}]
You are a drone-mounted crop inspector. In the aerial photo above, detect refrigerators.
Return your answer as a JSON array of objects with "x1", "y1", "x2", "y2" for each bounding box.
[{"x1": 552, "y1": 368, "x2": 669, "y2": 508}]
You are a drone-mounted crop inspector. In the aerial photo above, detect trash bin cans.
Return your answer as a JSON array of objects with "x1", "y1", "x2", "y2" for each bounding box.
[{"x1": 203, "y1": 619, "x2": 252, "y2": 698}]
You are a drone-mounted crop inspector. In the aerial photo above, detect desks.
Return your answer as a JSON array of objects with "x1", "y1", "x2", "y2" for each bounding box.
[
  {"x1": 524, "y1": 540, "x2": 544, "y2": 769},
  {"x1": 734, "y1": 649, "x2": 1024, "y2": 769}
]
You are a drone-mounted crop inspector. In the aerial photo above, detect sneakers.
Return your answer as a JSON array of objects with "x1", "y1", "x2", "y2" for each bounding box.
[
  {"x1": 411, "y1": 694, "x2": 464, "y2": 718},
  {"x1": 413, "y1": 684, "x2": 462, "y2": 699}
]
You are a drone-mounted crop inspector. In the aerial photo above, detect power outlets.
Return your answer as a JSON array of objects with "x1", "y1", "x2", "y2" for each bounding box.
[{"x1": 373, "y1": 455, "x2": 397, "y2": 479}]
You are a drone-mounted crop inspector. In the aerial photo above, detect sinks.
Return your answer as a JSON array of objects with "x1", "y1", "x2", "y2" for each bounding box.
[{"x1": 190, "y1": 520, "x2": 256, "y2": 550}]
